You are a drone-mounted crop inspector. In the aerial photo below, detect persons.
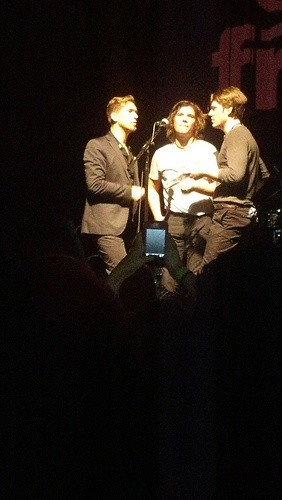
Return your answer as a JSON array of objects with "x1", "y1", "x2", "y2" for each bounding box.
[
  {"x1": 150, "y1": 99, "x2": 219, "y2": 298},
  {"x1": 1, "y1": 234, "x2": 282, "y2": 500},
  {"x1": 80, "y1": 92, "x2": 147, "y2": 276},
  {"x1": 181, "y1": 85, "x2": 272, "y2": 272}
]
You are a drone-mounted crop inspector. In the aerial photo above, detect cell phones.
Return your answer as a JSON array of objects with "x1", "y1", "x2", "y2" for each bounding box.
[{"x1": 143, "y1": 221, "x2": 168, "y2": 268}]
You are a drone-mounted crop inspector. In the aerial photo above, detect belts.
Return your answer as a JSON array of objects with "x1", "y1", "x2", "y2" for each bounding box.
[{"x1": 213, "y1": 201, "x2": 249, "y2": 210}]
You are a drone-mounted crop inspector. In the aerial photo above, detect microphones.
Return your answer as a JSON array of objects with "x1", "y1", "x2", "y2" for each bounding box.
[{"x1": 155, "y1": 118, "x2": 169, "y2": 135}]
